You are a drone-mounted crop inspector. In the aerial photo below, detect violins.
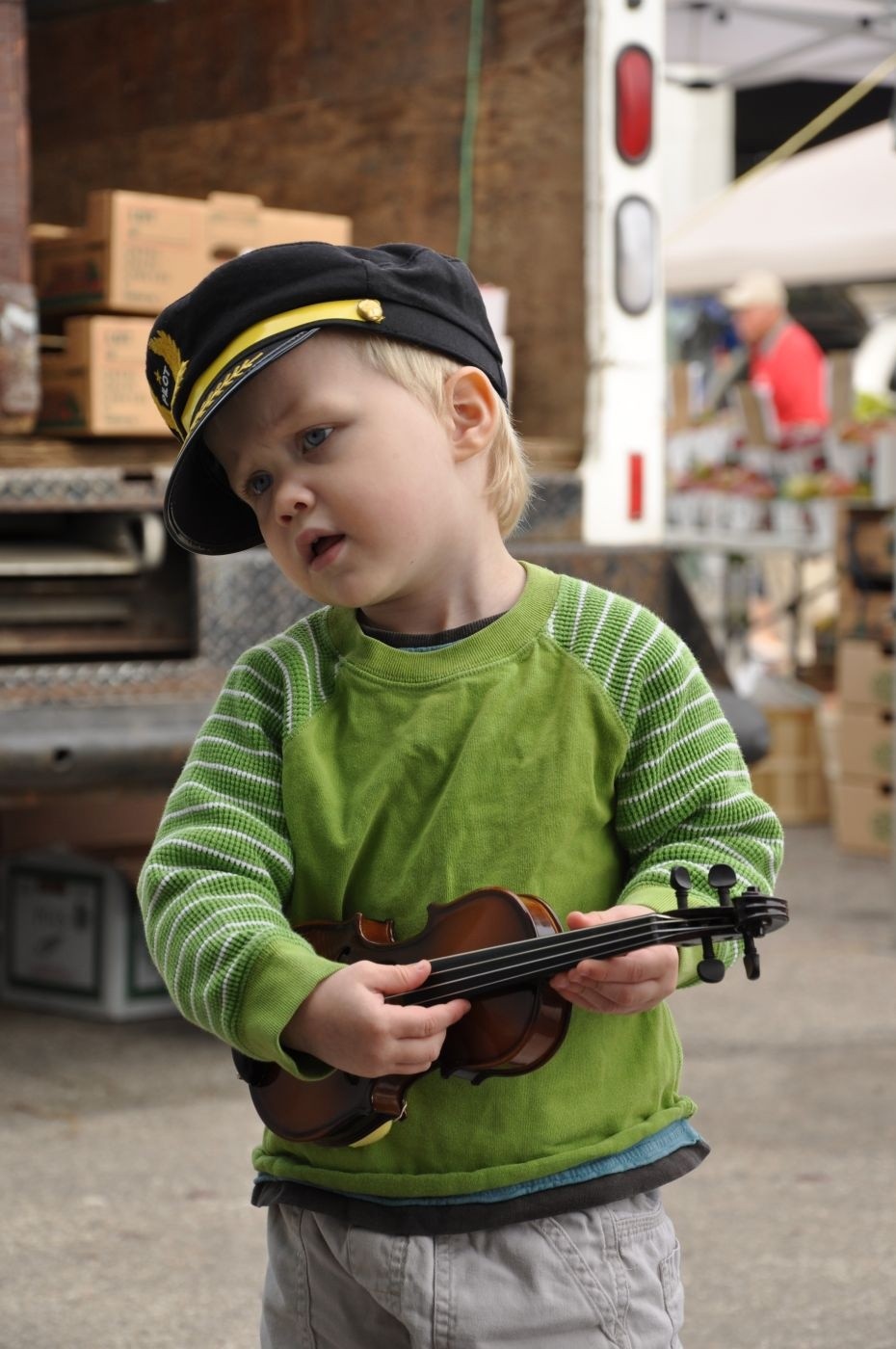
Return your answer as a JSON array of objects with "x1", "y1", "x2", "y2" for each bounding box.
[{"x1": 232, "y1": 864, "x2": 787, "y2": 1162}]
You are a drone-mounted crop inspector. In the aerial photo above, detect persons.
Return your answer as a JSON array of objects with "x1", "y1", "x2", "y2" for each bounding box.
[
  {"x1": 721, "y1": 268, "x2": 832, "y2": 661},
  {"x1": 132, "y1": 240, "x2": 786, "y2": 1348}
]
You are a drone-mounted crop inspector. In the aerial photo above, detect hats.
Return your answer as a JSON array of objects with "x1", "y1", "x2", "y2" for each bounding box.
[
  {"x1": 145, "y1": 240, "x2": 508, "y2": 557},
  {"x1": 719, "y1": 272, "x2": 788, "y2": 311}
]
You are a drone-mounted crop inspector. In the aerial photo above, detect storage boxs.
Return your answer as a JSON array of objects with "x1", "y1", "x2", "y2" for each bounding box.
[
  {"x1": 41, "y1": 316, "x2": 170, "y2": 435},
  {"x1": 749, "y1": 500, "x2": 896, "y2": 862},
  {"x1": 32, "y1": 190, "x2": 352, "y2": 314},
  {"x1": 0, "y1": 849, "x2": 178, "y2": 1023}
]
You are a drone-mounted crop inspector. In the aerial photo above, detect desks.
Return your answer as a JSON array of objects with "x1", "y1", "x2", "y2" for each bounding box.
[{"x1": 512, "y1": 530, "x2": 834, "y2": 674}]
